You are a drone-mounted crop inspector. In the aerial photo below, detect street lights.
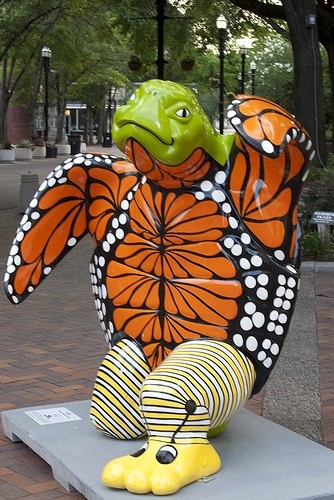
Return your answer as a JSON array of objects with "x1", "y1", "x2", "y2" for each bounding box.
[
  {"x1": 216, "y1": 14, "x2": 228, "y2": 135},
  {"x1": 65, "y1": 110, "x2": 71, "y2": 134},
  {"x1": 241, "y1": 44, "x2": 248, "y2": 94},
  {"x1": 41, "y1": 46, "x2": 52, "y2": 143},
  {"x1": 250, "y1": 61, "x2": 257, "y2": 95}
]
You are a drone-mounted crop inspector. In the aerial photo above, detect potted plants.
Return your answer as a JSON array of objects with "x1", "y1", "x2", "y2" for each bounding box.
[
  {"x1": 32, "y1": 139, "x2": 46, "y2": 158},
  {"x1": 15, "y1": 137, "x2": 35, "y2": 159},
  {"x1": 0, "y1": 141, "x2": 15, "y2": 163}
]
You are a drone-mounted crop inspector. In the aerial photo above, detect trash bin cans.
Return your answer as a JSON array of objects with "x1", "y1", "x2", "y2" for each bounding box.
[
  {"x1": 67, "y1": 135, "x2": 81, "y2": 155},
  {"x1": 102, "y1": 133, "x2": 112, "y2": 147}
]
